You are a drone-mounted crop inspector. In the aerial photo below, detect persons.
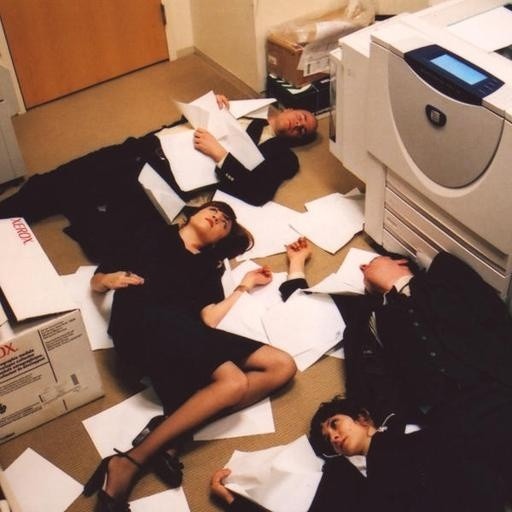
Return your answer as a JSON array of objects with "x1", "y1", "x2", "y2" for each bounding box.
[
  {"x1": 508, "y1": 505, "x2": 511, "y2": 507},
  {"x1": 83, "y1": 201, "x2": 297, "y2": 512},
  {"x1": 280, "y1": 235, "x2": 512, "y2": 451},
  {"x1": 209, "y1": 396, "x2": 511, "y2": 512},
  {"x1": 0, "y1": 95, "x2": 317, "y2": 226}
]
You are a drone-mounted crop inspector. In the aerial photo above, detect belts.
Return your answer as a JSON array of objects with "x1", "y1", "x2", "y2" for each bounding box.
[{"x1": 156, "y1": 147, "x2": 167, "y2": 160}]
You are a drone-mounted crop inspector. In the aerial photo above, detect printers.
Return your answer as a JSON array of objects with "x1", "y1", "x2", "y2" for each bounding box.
[{"x1": 328, "y1": 2, "x2": 512, "y2": 300}]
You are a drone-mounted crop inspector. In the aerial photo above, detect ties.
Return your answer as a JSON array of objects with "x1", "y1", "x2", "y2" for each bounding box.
[{"x1": 221, "y1": 118, "x2": 269, "y2": 170}]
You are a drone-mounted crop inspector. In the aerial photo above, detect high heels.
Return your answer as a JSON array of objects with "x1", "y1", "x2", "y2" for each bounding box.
[
  {"x1": 132, "y1": 415, "x2": 183, "y2": 488},
  {"x1": 83, "y1": 447, "x2": 142, "y2": 512}
]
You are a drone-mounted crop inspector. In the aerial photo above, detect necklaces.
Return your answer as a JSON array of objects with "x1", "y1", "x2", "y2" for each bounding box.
[{"x1": 180, "y1": 226, "x2": 195, "y2": 251}]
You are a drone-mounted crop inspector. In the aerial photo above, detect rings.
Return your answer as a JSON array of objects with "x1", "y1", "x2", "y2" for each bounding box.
[{"x1": 125, "y1": 271, "x2": 132, "y2": 277}]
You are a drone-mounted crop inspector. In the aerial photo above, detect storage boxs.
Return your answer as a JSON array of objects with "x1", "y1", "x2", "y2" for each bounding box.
[
  {"x1": 267, "y1": 0, "x2": 374, "y2": 87},
  {"x1": 0, "y1": 217, "x2": 106, "y2": 442},
  {"x1": 267, "y1": 72, "x2": 331, "y2": 110}
]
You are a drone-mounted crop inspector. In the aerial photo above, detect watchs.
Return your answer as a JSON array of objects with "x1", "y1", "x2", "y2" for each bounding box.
[{"x1": 233, "y1": 285, "x2": 252, "y2": 294}]
